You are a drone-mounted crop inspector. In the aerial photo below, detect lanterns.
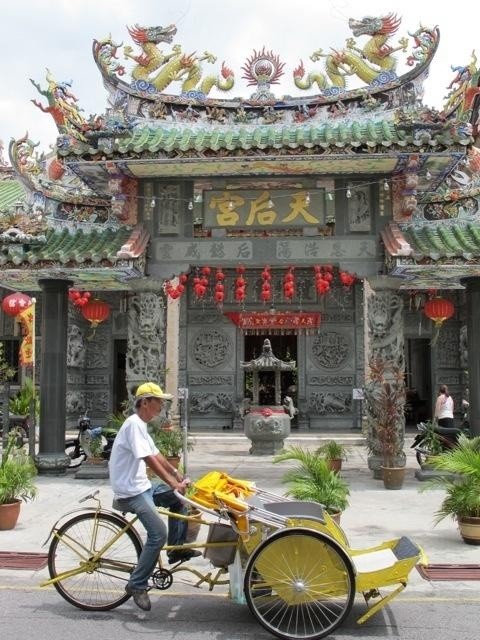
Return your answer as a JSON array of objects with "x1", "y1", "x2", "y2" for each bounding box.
[
  {"x1": 1, "y1": 292, "x2": 33, "y2": 340},
  {"x1": 68, "y1": 291, "x2": 110, "y2": 339},
  {"x1": 424, "y1": 288, "x2": 455, "y2": 344},
  {"x1": 165, "y1": 265, "x2": 353, "y2": 303}
]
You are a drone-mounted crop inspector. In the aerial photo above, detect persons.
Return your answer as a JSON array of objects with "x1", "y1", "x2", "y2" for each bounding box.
[
  {"x1": 110, "y1": 382, "x2": 202, "y2": 611},
  {"x1": 435, "y1": 385, "x2": 454, "y2": 429}
]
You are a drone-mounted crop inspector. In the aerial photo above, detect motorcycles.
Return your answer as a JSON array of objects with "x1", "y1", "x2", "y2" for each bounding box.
[
  {"x1": 0, "y1": 402, "x2": 30, "y2": 450},
  {"x1": 410, "y1": 422, "x2": 474, "y2": 470},
  {"x1": 65, "y1": 409, "x2": 117, "y2": 468}
]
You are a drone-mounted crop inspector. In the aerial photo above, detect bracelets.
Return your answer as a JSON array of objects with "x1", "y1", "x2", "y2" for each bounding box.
[{"x1": 171, "y1": 469, "x2": 177, "y2": 476}]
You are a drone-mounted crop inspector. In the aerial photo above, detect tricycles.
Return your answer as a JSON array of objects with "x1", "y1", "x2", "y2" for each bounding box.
[{"x1": 39, "y1": 469, "x2": 425, "y2": 639}]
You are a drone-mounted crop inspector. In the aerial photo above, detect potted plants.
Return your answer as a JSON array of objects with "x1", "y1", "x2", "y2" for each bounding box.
[
  {"x1": 417, "y1": 431, "x2": 480, "y2": 546},
  {"x1": 158, "y1": 427, "x2": 197, "y2": 471},
  {"x1": 357, "y1": 382, "x2": 414, "y2": 490},
  {"x1": 270, "y1": 438, "x2": 349, "y2": 527},
  {"x1": 0, "y1": 425, "x2": 40, "y2": 532}
]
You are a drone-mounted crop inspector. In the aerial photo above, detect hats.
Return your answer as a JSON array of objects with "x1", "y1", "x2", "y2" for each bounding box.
[{"x1": 135, "y1": 382, "x2": 174, "y2": 400}]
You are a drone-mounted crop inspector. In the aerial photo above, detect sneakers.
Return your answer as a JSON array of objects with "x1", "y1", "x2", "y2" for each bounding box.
[
  {"x1": 125, "y1": 584, "x2": 151, "y2": 610},
  {"x1": 169, "y1": 549, "x2": 202, "y2": 564}
]
